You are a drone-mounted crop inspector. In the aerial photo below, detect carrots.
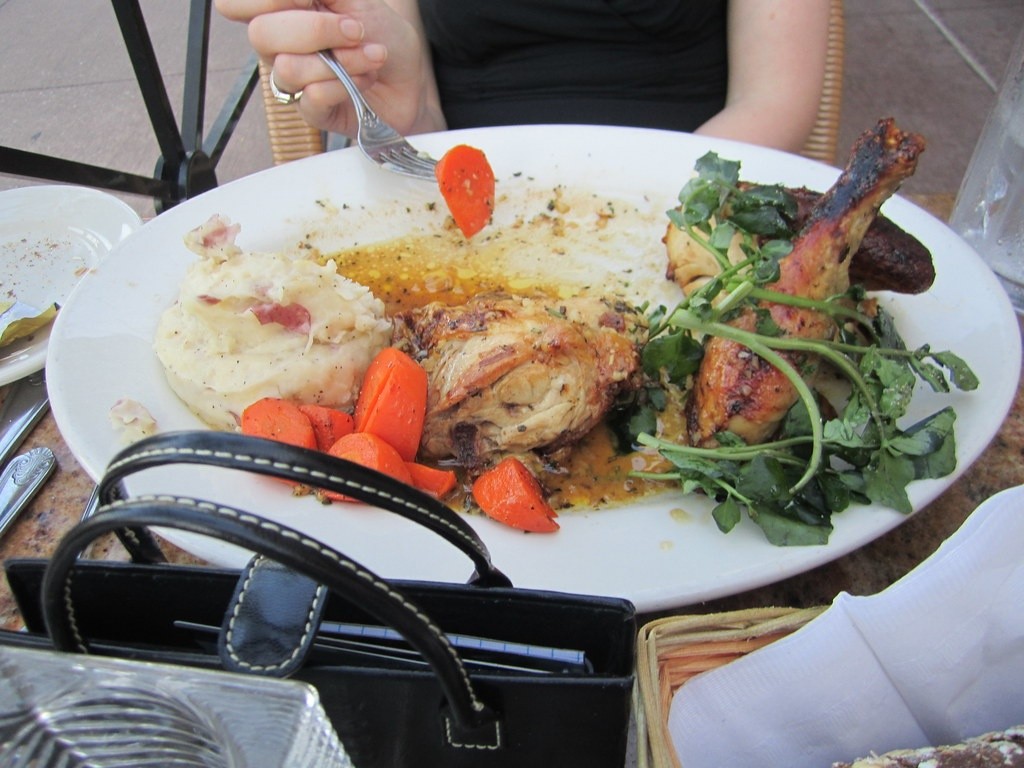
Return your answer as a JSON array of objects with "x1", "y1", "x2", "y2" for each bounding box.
[
  {"x1": 472, "y1": 456, "x2": 560, "y2": 533},
  {"x1": 436, "y1": 145, "x2": 495, "y2": 240},
  {"x1": 239, "y1": 345, "x2": 456, "y2": 502}
]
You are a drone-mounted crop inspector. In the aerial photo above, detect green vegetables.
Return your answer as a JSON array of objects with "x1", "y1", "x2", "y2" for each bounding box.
[{"x1": 607, "y1": 151, "x2": 981, "y2": 547}]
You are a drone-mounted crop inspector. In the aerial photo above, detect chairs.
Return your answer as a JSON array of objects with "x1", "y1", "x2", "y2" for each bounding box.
[{"x1": 257, "y1": 0, "x2": 846, "y2": 166}]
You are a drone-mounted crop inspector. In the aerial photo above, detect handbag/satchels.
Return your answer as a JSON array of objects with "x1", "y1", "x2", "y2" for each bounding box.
[{"x1": 0, "y1": 431, "x2": 637, "y2": 768}]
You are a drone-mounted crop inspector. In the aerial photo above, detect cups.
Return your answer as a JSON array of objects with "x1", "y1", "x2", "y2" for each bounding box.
[{"x1": 945, "y1": 14, "x2": 1024, "y2": 328}]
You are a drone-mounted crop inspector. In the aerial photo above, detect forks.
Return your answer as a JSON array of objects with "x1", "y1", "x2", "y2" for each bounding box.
[{"x1": 315, "y1": 48, "x2": 438, "y2": 182}]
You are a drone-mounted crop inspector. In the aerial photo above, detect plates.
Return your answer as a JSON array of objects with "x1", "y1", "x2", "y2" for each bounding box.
[
  {"x1": 43, "y1": 123, "x2": 1023, "y2": 613},
  {"x1": 0, "y1": 185, "x2": 146, "y2": 387}
]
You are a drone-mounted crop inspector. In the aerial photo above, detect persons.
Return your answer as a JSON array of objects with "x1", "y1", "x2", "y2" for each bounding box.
[{"x1": 212, "y1": 0, "x2": 832, "y2": 157}]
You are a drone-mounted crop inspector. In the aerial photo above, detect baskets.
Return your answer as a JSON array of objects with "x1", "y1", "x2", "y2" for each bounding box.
[{"x1": 639, "y1": 603, "x2": 829, "y2": 768}]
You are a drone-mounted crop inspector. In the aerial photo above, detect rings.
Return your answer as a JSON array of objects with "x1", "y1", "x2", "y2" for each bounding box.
[{"x1": 268, "y1": 69, "x2": 303, "y2": 105}]
